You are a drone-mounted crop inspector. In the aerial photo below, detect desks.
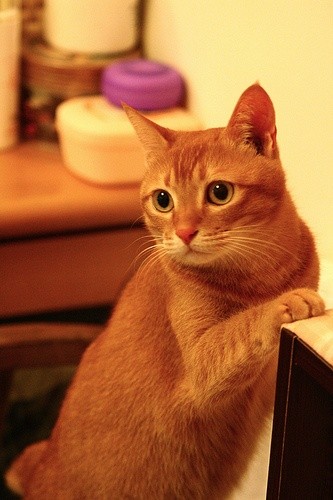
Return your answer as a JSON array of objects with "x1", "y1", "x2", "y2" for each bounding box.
[{"x1": 0, "y1": 131, "x2": 176, "y2": 317}]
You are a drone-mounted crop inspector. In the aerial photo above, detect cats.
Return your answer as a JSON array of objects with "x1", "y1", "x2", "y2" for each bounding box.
[{"x1": 4, "y1": 81, "x2": 325, "y2": 500}]
